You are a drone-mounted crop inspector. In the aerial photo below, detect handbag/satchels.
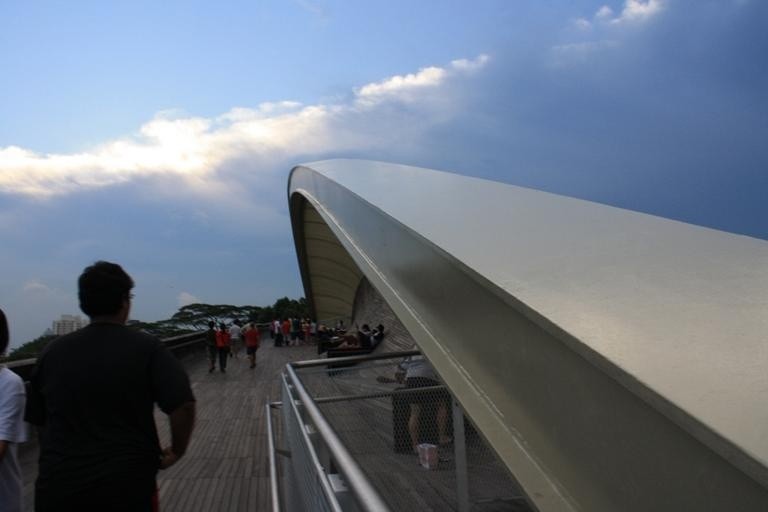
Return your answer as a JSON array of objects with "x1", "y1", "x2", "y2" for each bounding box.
[{"x1": 224, "y1": 346, "x2": 231, "y2": 353}]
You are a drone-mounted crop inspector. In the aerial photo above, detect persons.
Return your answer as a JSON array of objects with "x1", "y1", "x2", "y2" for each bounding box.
[
  {"x1": 393, "y1": 342, "x2": 452, "y2": 456},
  {"x1": 20, "y1": 257, "x2": 198, "y2": 511},
  {"x1": 239, "y1": 321, "x2": 258, "y2": 359},
  {"x1": 242, "y1": 324, "x2": 259, "y2": 370},
  {"x1": 0, "y1": 308, "x2": 29, "y2": 510},
  {"x1": 203, "y1": 319, "x2": 215, "y2": 372},
  {"x1": 227, "y1": 320, "x2": 241, "y2": 359},
  {"x1": 214, "y1": 323, "x2": 231, "y2": 374},
  {"x1": 268, "y1": 316, "x2": 385, "y2": 358}
]
